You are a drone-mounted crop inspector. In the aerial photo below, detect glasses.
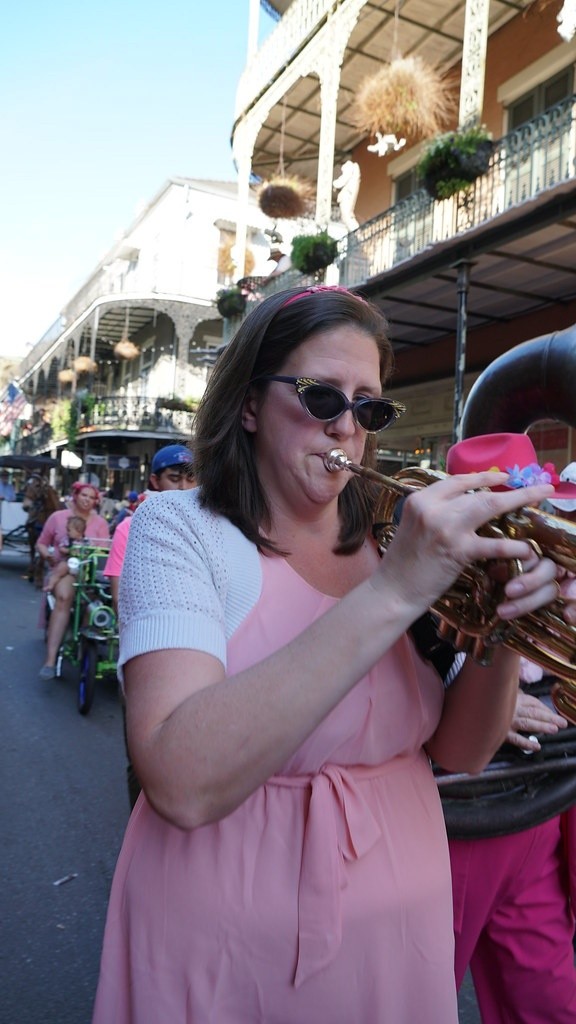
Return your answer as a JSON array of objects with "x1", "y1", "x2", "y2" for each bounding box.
[{"x1": 265, "y1": 375, "x2": 407, "y2": 434}]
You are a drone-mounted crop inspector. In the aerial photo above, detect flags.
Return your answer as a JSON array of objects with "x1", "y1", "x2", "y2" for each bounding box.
[{"x1": 0, "y1": 382, "x2": 29, "y2": 437}]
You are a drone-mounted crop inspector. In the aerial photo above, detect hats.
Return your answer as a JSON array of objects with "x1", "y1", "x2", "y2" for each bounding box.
[
  {"x1": 447, "y1": 433, "x2": 576, "y2": 499},
  {"x1": 128, "y1": 493, "x2": 138, "y2": 502},
  {"x1": 147, "y1": 445, "x2": 195, "y2": 491},
  {"x1": 546, "y1": 462, "x2": 576, "y2": 512}
]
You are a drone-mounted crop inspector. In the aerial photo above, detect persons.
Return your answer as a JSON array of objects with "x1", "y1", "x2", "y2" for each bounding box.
[
  {"x1": 89, "y1": 285, "x2": 557, "y2": 1024},
  {"x1": 423, "y1": 432, "x2": 576, "y2": 1024},
  {"x1": 263, "y1": 248, "x2": 307, "y2": 286},
  {"x1": 103, "y1": 445, "x2": 198, "y2": 814},
  {"x1": 0, "y1": 469, "x2": 146, "y2": 680}
]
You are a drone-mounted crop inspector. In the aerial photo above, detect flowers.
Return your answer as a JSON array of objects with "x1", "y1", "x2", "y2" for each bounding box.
[{"x1": 485, "y1": 459, "x2": 562, "y2": 494}]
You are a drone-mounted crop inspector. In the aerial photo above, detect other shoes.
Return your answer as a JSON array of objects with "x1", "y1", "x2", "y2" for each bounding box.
[{"x1": 40, "y1": 665, "x2": 56, "y2": 681}]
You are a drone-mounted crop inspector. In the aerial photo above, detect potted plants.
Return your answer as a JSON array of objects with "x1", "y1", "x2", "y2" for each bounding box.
[
  {"x1": 214, "y1": 286, "x2": 251, "y2": 318},
  {"x1": 412, "y1": 122, "x2": 499, "y2": 202},
  {"x1": 287, "y1": 225, "x2": 341, "y2": 277},
  {"x1": 77, "y1": 393, "x2": 97, "y2": 415}
]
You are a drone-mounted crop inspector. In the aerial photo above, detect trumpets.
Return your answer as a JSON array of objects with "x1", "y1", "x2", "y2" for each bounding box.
[{"x1": 322, "y1": 444, "x2": 576, "y2": 727}]
